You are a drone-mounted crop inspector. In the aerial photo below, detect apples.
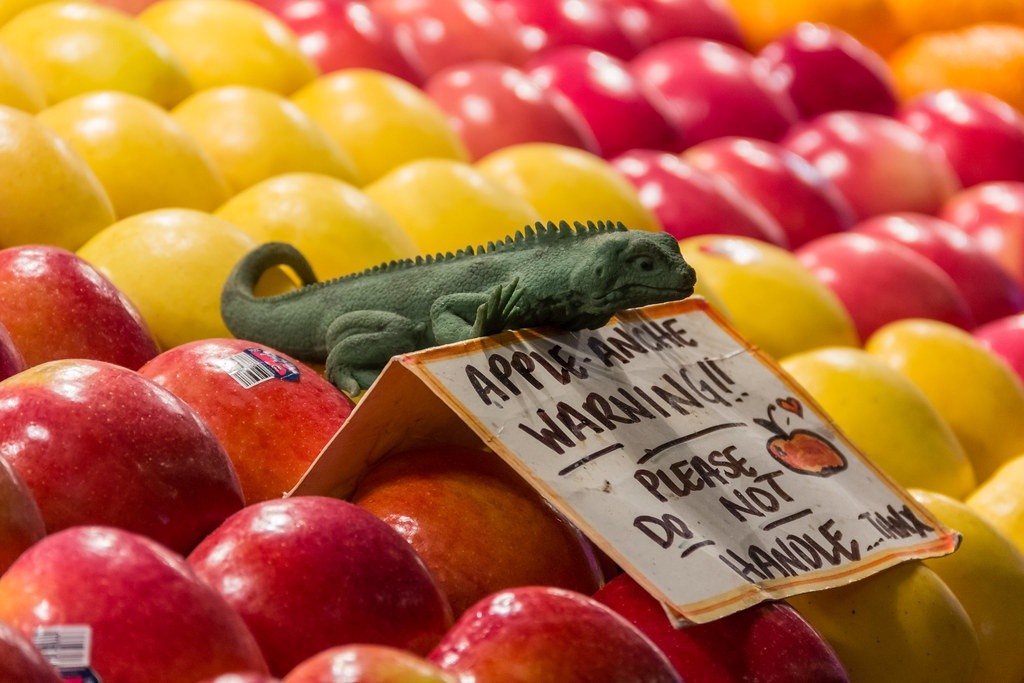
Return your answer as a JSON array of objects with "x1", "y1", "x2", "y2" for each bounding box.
[{"x1": 0, "y1": 0, "x2": 1024, "y2": 683}]
